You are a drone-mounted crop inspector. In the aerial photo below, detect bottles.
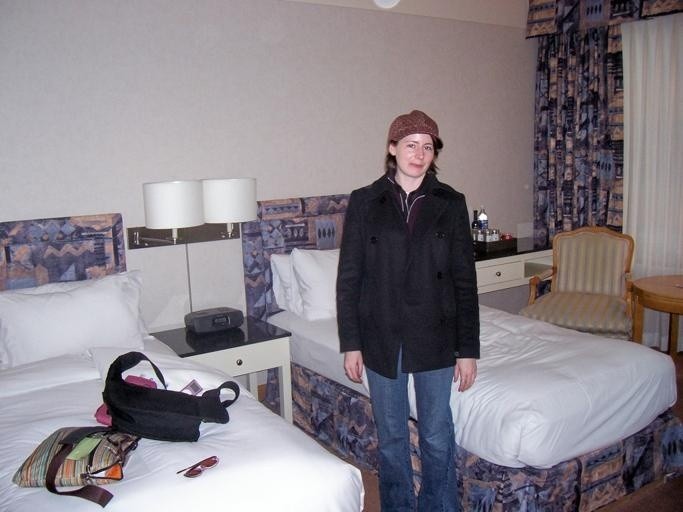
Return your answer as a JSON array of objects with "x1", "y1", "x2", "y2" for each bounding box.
[{"x1": 472, "y1": 205, "x2": 513, "y2": 242}]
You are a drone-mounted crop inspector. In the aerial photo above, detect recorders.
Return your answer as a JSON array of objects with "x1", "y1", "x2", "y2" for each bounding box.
[{"x1": 184, "y1": 306, "x2": 244, "y2": 334}]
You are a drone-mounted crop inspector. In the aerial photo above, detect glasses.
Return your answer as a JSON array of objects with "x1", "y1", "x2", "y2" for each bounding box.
[{"x1": 177, "y1": 456, "x2": 219, "y2": 478}]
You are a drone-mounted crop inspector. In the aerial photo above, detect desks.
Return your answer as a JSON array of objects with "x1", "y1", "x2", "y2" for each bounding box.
[{"x1": 631, "y1": 274, "x2": 682, "y2": 373}]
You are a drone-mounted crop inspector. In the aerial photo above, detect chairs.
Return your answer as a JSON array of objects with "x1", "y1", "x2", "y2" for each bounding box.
[{"x1": 519, "y1": 226, "x2": 631, "y2": 339}]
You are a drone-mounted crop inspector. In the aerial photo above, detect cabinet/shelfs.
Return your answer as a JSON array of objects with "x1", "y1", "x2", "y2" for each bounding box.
[{"x1": 475, "y1": 233, "x2": 554, "y2": 295}]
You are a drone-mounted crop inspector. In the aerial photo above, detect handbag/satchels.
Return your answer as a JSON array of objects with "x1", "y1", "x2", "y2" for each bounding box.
[
  {"x1": 101, "y1": 367, "x2": 202, "y2": 442},
  {"x1": 12, "y1": 425, "x2": 143, "y2": 488}
]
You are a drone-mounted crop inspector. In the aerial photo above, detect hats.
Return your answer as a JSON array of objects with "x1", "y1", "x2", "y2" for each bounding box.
[{"x1": 387, "y1": 110, "x2": 444, "y2": 152}]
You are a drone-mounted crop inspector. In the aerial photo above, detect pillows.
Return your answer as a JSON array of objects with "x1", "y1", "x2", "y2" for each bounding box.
[
  {"x1": 5, "y1": 268, "x2": 150, "y2": 339},
  {"x1": 268, "y1": 253, "x2": 303, "y2": 314},
  {"x1": 291, "y1": 246, "x2": 342, "y2": 321},
  {"x1": 0, "y1": 277, "x2": 143, "y2": 367}
]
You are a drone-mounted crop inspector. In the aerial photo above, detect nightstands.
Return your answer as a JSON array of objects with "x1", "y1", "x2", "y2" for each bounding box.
[{"x1": 150, "y1": 313, "x2": 294, "y2": 425}]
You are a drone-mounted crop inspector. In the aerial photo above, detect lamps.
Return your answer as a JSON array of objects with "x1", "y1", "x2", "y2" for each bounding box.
[
  {"x1": 132, "y1": 177, "x2": 204, "y2": 245},
  {"x1": 203, "y1": 176, "x2": 258, "y2": 239}
]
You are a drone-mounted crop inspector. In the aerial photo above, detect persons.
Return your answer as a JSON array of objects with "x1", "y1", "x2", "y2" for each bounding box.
[{"x1": 335, "y1": 110, "x2": 481, "y2": 512}]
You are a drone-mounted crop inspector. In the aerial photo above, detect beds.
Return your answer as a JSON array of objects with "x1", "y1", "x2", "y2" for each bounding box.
[
  {"x1": 241, "y1": 193, "x2": 683, "y2": 512},
  {"x1": 0, "y1": 213, "x2": 365, "y2": 511}
]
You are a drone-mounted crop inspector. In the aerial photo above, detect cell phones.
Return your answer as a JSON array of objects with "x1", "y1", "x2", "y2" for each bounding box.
[{"x1": 180, "y1": 380, "x2": 203, "y2": 396}]
